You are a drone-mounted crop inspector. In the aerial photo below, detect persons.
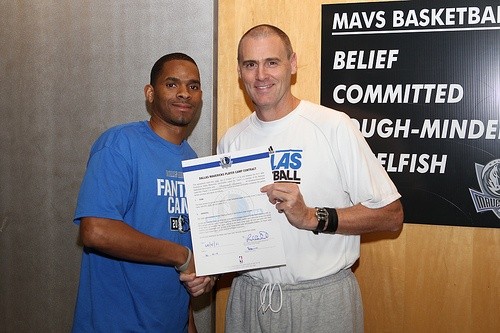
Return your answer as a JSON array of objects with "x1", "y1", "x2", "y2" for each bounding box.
[
  {"x1": 69, "y1": 52, "x2": 212, "y2": 333},
  {"x1": 180, "y1": 25, "x2": 406, "y2": 333}
]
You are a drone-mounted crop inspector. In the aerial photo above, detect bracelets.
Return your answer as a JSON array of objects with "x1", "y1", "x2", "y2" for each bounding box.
[
  {"x1": 321, "y1": 206, "x2": 339, "y2": 235},
  {"x1": 173, "y1": 246, "x2": 192, "y2": 272}
]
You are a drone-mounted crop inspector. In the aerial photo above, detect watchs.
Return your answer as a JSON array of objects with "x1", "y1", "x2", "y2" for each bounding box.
[{"x1": 313, "y1": 206, "x2": 328, "y2": 236}]
